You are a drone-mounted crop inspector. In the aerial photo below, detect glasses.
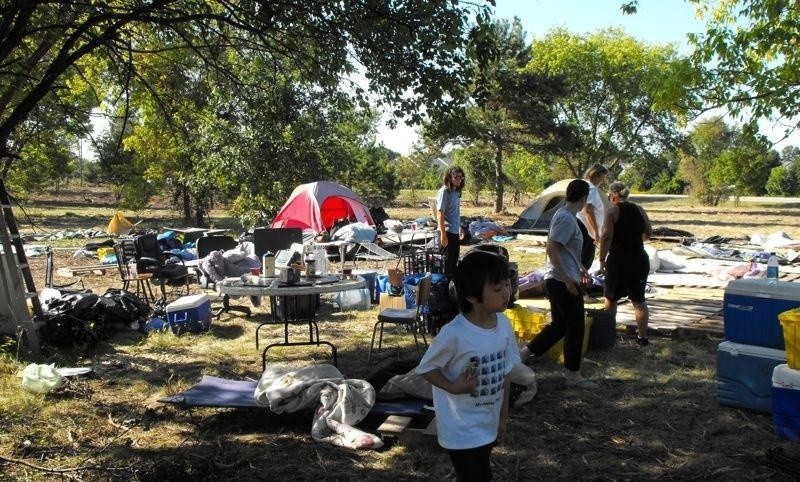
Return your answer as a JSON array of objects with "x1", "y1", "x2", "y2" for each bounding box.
[{"x1": 453, "y1": 176, "x2": 464, "y2": 181}]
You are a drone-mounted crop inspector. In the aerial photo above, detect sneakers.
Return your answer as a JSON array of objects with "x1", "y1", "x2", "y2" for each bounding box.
[
  {"x1": 519, "y1": 347, "x2": 541, "y2": 367},
  {"x1": 636, "y1": 332, "x2": 649, "y2": 346},
  {"x1": 566, "y1": 377, "x2": 599, "y2": 390}
]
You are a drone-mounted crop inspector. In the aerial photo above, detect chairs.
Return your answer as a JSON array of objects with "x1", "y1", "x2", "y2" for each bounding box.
[
  {"x1": 368, "y1": 271, "x2": 435, "y2": 368},
  {"x1": 194, "y1": 234, "x2": 253, "y2": 322},
  {"x1": 134, "y1": 232, "x2": 195, "y2": 303},
  {"x1": 121, "y1": 239, "x2": 139, "y2": 275},
  {"x1": 114, "y1": 243, "x2": 157, "y2": 307}
]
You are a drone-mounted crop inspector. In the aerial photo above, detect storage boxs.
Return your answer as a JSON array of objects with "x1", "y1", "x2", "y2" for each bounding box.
[
  {"x1": 166, "y1": 295, "x2": 215, "y2": 339},
  {"x1": 716, "y1": 339, "x2": 790, "y2": 417},
  {"x1": 721, "y1": 277, "x2": 800, "y2": 352},
  {"x1": 348, "y1": 270, "x2": 377, "y2": 305},
  {"x1": 537, "y1": 314, "x2": 594, "y2": 366},
  {"x1": 776, "y1": 305, "x2": 800, "y2": 370},
  {"x1": 769, "y1": 364, "x2": 800, "y2": 444},
  {"x1": 379, "y1": 291, "x2": 407, "y2": 313}
]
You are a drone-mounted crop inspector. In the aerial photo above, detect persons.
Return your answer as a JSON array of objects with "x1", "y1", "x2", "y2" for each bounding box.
[
  {"x1": 436, "y1": 164, "x2": 465, "y2": 280},
  {"x1": 576, "y1": 162, "x2": 608, "y2": 272},
  {"x1": 414, "y1": 253, "x2": 522, "y2": 481},
  {"x1": 598, "y1": 181, "x2": 654, "y2": 346},
  {"x1": 519, "y1": 178, "x2": 600, "y2": 390}
]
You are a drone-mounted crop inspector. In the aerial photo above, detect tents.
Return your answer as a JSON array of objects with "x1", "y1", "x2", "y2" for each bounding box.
[
  {"x1": 271, "y1": 181, "x2": 377, "y2": 234},
  {"x1": 510, "y1": 180, "x2": 615, "y2": 239}
]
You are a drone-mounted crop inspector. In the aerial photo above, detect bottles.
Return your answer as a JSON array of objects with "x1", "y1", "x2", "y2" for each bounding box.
[{"x1": 766, "y1": 251, "x2": 779, "y2": 289}]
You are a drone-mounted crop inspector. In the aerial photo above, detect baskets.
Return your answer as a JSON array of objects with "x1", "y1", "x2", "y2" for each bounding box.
[
  {"x1": 404, "y1": 273, "x2": 449, "y2": 314},
  {"x1": 779, "y1": 308, "x2": 800, "y2": 369},
  {"x1": 546, "y1": 316, "x2": 592, "y2": 363},
  {"x1": 585, "y1": 308, "x2": 616, "y2": 351}
]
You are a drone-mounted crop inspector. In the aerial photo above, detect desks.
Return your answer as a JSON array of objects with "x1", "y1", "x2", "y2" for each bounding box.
[
  {"x1": 176, "y1": 258, "x2": 210, "y2": 297},
  {"x1": 217, "y1": 273, "x2": 368, "y2": 374}
]
